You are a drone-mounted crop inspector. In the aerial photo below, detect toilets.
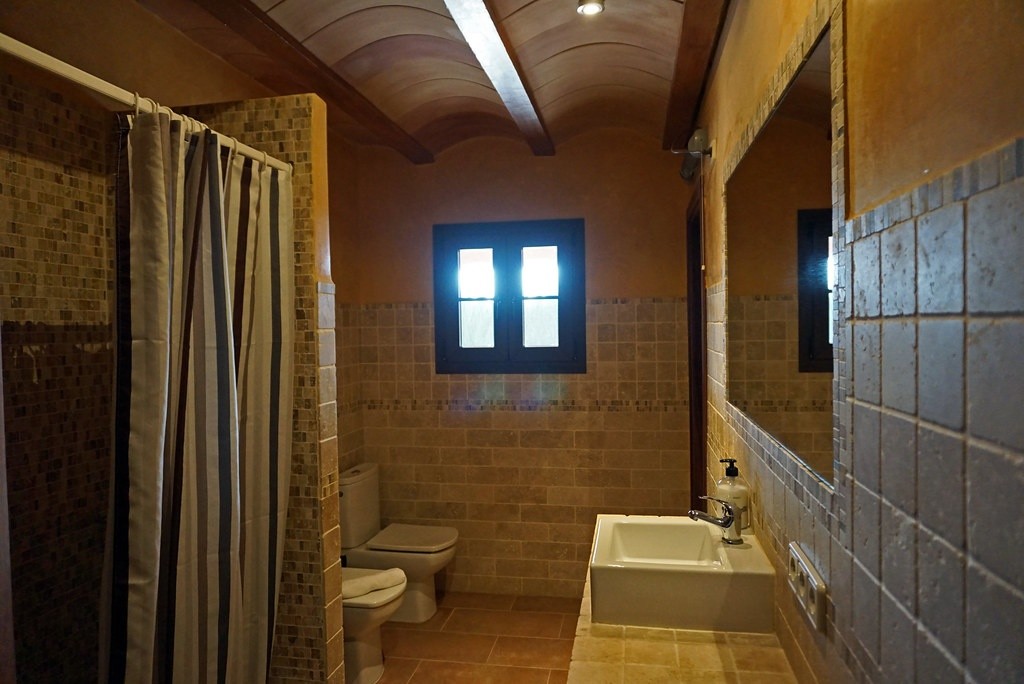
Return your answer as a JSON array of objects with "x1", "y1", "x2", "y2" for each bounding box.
[
  {"x1": 343, "y1": 567, "x2": 408, "y2": 684},
  {"x1": 339, "y1": 461, "x2": 459, "y2": 623}
]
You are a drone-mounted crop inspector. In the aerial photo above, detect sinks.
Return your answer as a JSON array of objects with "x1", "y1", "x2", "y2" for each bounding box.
[{"x1": 589, "y1": 518, "x2": 777, "y2": 632}]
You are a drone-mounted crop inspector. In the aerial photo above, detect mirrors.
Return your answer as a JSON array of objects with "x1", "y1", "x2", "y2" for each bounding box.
[{"x1": 726, "y1": 21, "x2": 834, "y2": 488}]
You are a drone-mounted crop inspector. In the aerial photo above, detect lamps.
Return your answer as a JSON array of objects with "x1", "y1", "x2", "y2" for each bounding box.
[{"x1": 577, "y1": 0, "x2": 605, "y2": 15}]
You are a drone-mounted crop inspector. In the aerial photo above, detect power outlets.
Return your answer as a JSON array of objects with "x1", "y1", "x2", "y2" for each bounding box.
[{"x1": 786, "y1": 541, "x2": 828, "y2": 638}]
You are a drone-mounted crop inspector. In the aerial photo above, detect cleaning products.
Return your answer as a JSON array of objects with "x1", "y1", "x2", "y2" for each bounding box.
[{"x1": 716, "y1": 458, "x2": 750, "y2": 530}]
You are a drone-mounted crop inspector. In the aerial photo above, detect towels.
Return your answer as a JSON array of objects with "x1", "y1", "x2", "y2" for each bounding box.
[{"x1": 342, "y1": 565, "x2": 406, "y2": 599}]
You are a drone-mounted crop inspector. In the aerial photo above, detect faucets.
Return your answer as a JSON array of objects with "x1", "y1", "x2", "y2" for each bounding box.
[{"x1": 688, "y1": 495, "x2": 742, "y2": 545}]
